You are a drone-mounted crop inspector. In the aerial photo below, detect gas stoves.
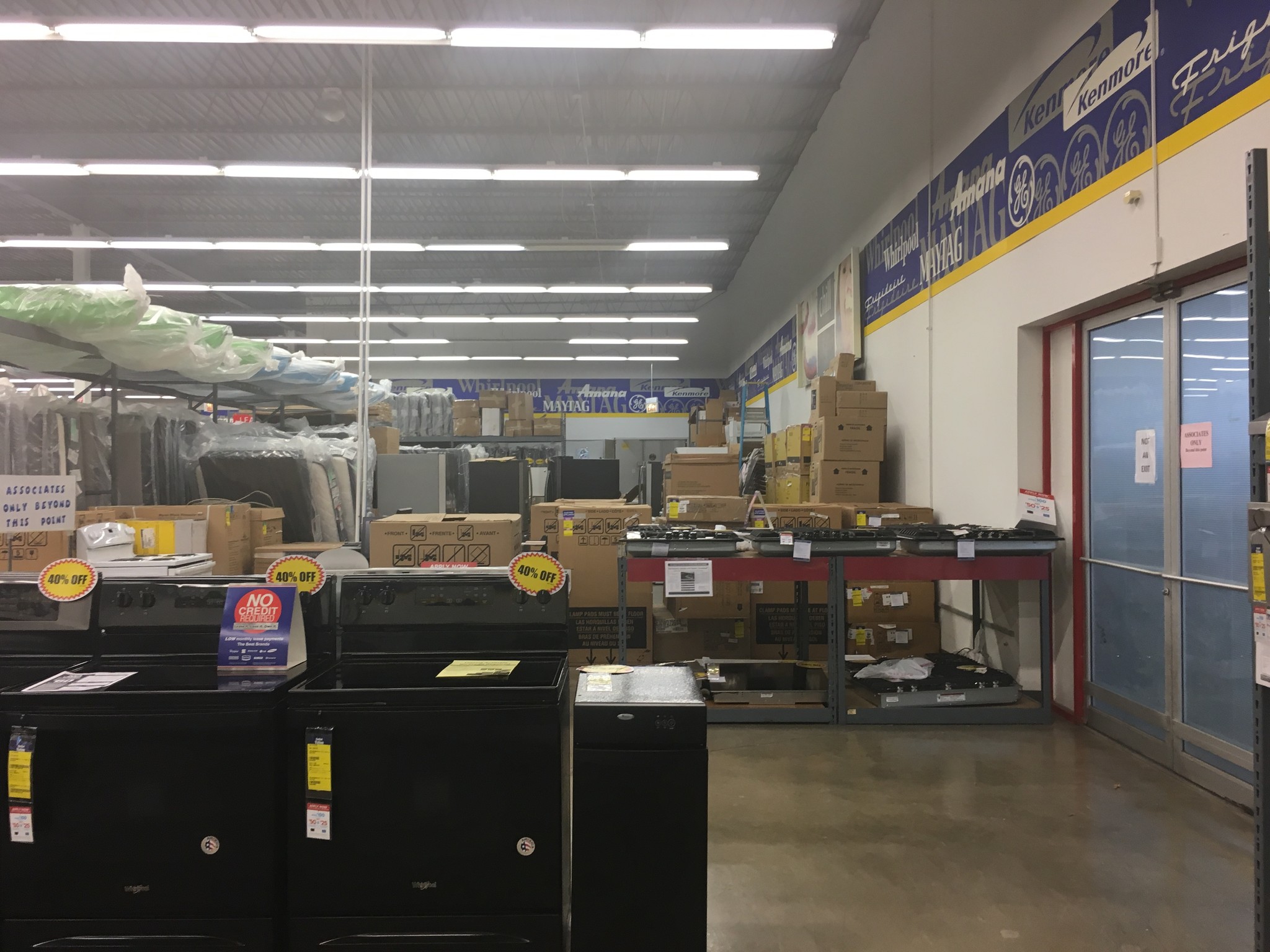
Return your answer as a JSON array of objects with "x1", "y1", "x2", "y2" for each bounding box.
[
  {"x1": 77, "y1": 522, "x2": 213, "y2": 567},
  {"x1": 619, "y1": 523, "x2": 1064, "y2": 557}
]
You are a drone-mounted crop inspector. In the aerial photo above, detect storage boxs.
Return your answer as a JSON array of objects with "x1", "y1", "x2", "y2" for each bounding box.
[
  {"x1": 453, "y1": 391, "x2": 560, "y2": 436},
  {"x1": 369, "y1": 426, "x2": 400, "y2": 454},
  {"x1": 1, "y1": 503, "x2": 363, "y2": 577},
  {"x1": 368, "y1": 513, "x2": 523, "y2": 567},
  {"x1": 651, "y1": 352, "x2": 941, "y2": 674},
  {"x1": 530, "y1": 498, "x2": 652, "y2": 668}
]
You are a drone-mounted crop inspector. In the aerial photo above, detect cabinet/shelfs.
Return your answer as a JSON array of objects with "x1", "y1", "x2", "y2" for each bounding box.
[
  {"x1": 836, "y1": 554, "x2": 1054, "y2": 725},
  {"x1": 617, "y1": 543, "x2": 836, "y2": 725}
]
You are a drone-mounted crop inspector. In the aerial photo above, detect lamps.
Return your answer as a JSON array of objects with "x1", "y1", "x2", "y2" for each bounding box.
[
  {"x1": 0, "y1": 235, "x2": 730, "y2": 252},
  {"x1": 0, "y1": 158, "x2": 760, "y2": 182},
  {"x1": 0, "y1": 280, "x2": 714, "y2": 294},
  {"x1": 197, "y1": 313, "x2": 699, "y2": 323},
  {"x1": 0, "y1": 14, "x2": 836, "y2": 51}
]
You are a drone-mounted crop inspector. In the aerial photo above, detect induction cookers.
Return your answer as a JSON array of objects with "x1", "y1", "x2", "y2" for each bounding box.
[{"x1": 0, "y1": 566, "x2": 567, "y2": 706}]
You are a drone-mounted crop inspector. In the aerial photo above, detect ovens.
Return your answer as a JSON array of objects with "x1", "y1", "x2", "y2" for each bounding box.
[
  {"x1": 0, "y1": 712, "x2": 565, "y2": 952},
  {"x1": 167, "y1": 560, "x2": 216, "y2": 576}
]
